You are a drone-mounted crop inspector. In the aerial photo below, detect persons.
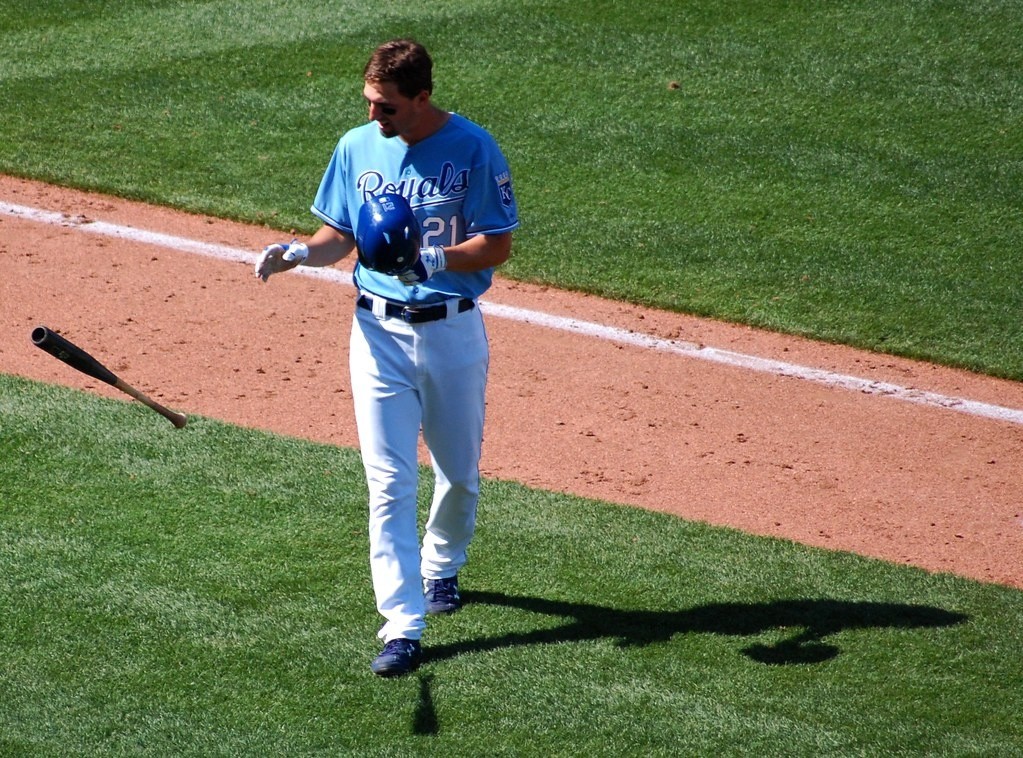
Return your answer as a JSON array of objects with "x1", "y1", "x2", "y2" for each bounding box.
[{"x1": 256, "y1": 39, "x2": 520, "y2": 676}]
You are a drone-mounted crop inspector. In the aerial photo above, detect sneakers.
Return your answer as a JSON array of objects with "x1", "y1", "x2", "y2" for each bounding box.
[
  {"x1": 370, "y1": 638, "x2": 421, "y2": 675},
  {"x1": 423, "y1": 574, "x2": 459, "y2": 613}
]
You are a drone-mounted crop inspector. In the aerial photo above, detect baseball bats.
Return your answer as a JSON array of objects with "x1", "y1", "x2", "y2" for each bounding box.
[{"x1": 31, "y1": 324, "x2": 190, "y2": 430}]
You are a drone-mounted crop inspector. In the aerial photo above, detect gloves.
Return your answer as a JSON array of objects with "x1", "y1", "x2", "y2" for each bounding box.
[
  {"x1": 255, "y1": 239, "x2": 308, "y2": 282},
  {"x1": 392, "y1": 246, "x2": 448, "y2": 286}
]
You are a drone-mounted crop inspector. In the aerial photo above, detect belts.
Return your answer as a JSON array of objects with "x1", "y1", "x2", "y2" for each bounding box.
[{"x1": 355, "y1": 294, "x2": 475, "y2": 323}]
